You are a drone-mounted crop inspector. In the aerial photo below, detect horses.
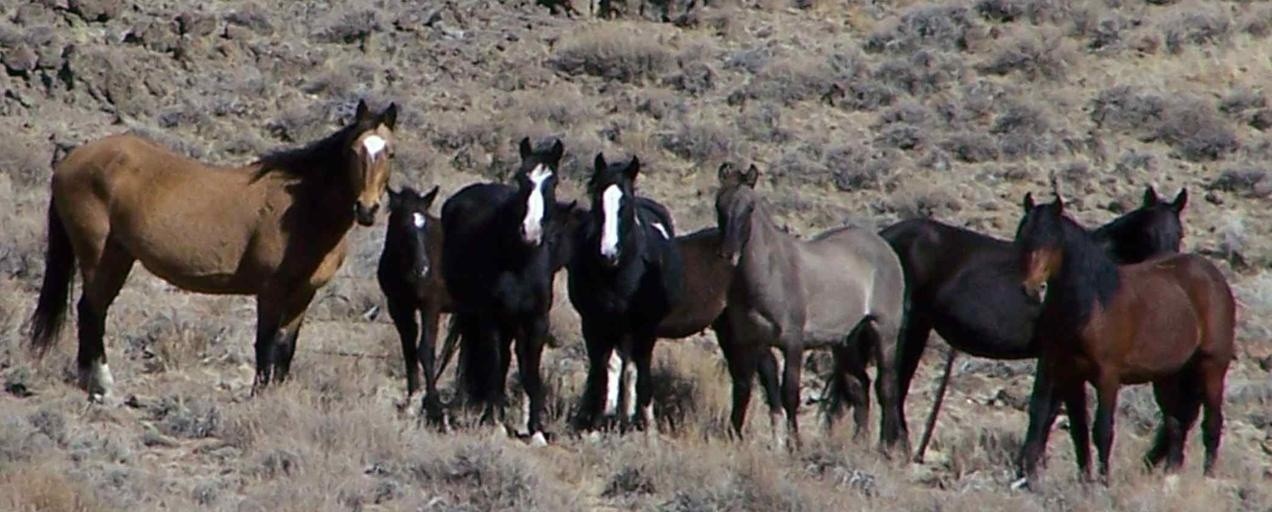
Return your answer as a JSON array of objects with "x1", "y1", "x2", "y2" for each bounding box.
[
  {"x1": 1013, "y1": 193, "x2": 1237, "y2": 496},
  {"x1": 375, "y1": 136, "x2": 757, "y2": 448},
  {"x1": 814, "y1": 185, "x2": 1190, "y2": 462},
  {"x1": 714, "y1": 158, "x2": 908, "y2": 464},
  {"x1": 15, "y1": 94, "x2": 402, "y2": 407}
]
